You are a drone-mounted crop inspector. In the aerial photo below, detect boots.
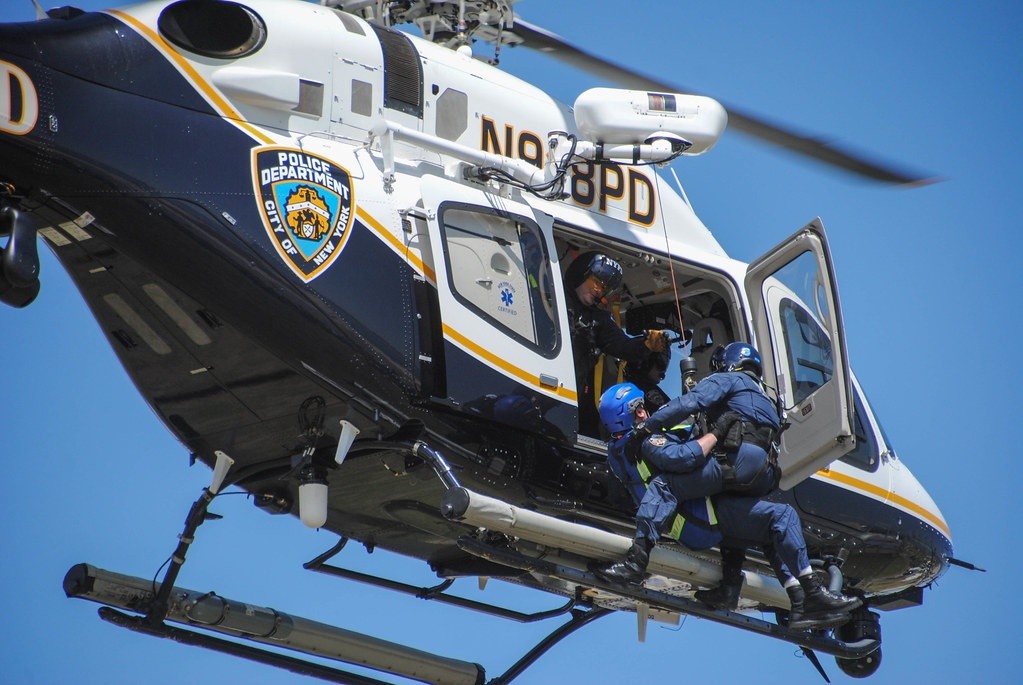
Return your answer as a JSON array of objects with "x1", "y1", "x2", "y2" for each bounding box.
[
  {"x1": 694, "y1": 571, "x2": 744, "y2": 609},
  {"x1": 786, "y1": 584, "x2": 850, "y2": 631},
  {"x1": 797, "y1": 572, "x2": 863, "y2": 615},
  {"x1": 587, "y1": 537, "x2": 654, "y2": 586}
]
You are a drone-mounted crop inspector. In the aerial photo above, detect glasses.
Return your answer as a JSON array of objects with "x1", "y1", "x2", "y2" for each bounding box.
[{"x1": 655, "y1": 359, "x2": 666, "y2": 370}]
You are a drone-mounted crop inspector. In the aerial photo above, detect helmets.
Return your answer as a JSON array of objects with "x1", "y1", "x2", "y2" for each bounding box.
[
  {"x1": 716, "y1": 342, "x2": 762, "y2": 383},
  {"x1": 599, "y1": 383, "x2": 644, "y2": 433},
  {"x1": 565, "y1": 251, "x2": 623, "y2": 296}
]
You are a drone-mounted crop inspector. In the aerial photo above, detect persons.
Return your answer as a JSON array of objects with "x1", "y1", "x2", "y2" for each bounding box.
[
  {"x1": 587, "y1": 341, "x2": 781, "y2": 609},
  {"x1": 597, "y1": 382, "x2": 863, "y2": 631},
  {"x1": 563, "y1": 251, "x2": 672, "y2": 439}
]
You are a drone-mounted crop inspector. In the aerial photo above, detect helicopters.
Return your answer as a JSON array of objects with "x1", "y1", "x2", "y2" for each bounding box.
[{"x1": 0, "y1": 0, "x2": 988, "y2": 685}]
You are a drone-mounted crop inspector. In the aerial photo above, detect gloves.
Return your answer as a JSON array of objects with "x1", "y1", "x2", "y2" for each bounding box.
[
  {"x1": 644, "y1": 330, "x2": 663, "y2": 352},
  {"x1": 624, "y1": 428, "x2": 650, "y2": 466},
  {"x1": 644, "y1": 329, "x2": 671, "y2": 345},
  {"x1": 709, "y1": 410, "x2": 740, "y2": 445}
]
